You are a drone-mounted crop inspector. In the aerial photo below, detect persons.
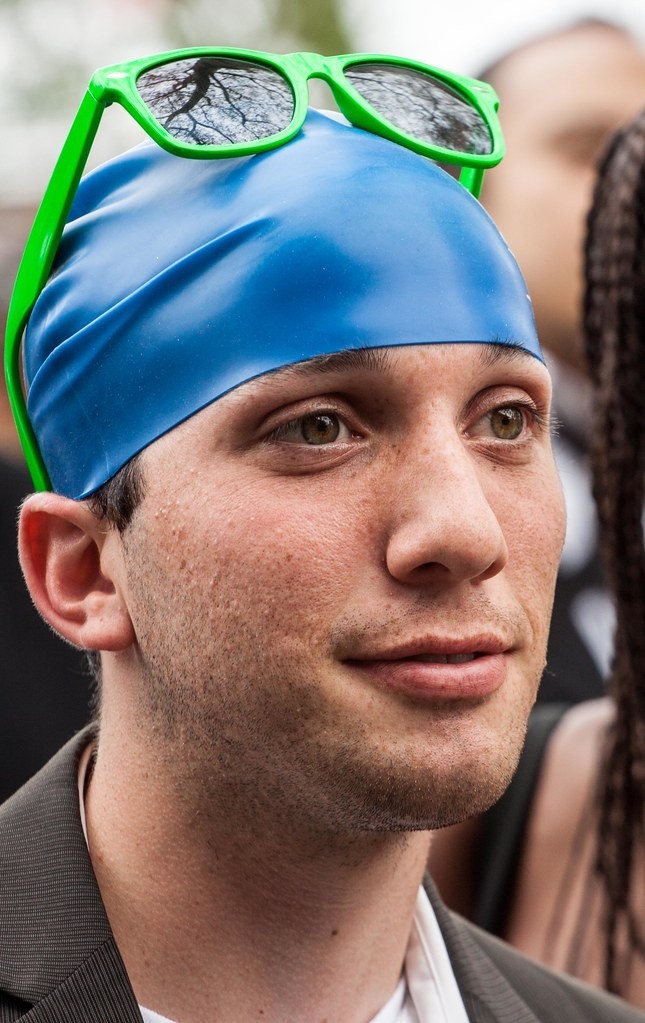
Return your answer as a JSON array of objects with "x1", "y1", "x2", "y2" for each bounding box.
[{"x1": 0, "y1": 13, "x2": 645, "y2": 1023}]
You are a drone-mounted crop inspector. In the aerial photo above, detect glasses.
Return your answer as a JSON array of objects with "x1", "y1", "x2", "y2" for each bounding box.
[{"x1": 4, "y1": 50, "x2": 505, "y2": 494}]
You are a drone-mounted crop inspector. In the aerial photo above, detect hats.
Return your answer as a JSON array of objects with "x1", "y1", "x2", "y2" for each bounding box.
[{"x1": 23, "y1": 111, "x2": 547, "y2": 505}]
